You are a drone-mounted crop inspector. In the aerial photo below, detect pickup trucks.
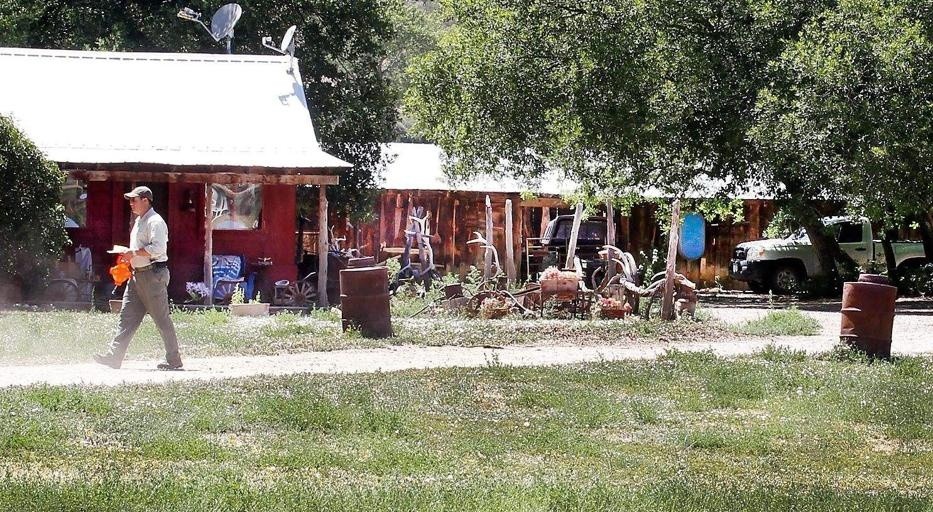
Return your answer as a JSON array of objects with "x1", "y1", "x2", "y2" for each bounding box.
[
  {"x1": 728, "y1": 214, "x2": 932, "y2": 297},
  {"x1": 535, "y1": 215, "x2": 642, "y2": 288}
]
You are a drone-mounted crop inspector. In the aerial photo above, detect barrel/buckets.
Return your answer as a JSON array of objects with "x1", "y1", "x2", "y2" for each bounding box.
[
  {"x1": 839, "y1": 281, "x2": 898, "y2": 361},
  {"x1": 339, "y1": 267, "x2": 394, "y2": 341}
]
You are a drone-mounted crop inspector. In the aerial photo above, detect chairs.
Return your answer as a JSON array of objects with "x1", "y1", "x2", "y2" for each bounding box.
[{"x1": 211, "y1": 254, "x2": 248, "y2": 304}]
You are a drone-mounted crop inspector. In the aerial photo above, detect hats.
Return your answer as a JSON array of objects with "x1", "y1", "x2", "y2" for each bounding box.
[{"x1": 123, "y1": 185, "x2": 154, "y2": 202}]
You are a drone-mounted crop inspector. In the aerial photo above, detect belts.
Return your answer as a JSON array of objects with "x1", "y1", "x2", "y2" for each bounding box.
[{"x1": 133, "y1": 261, "x2": 167, "y2": 273}]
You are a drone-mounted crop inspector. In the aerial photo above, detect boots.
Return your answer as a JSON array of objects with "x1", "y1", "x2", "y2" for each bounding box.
[
  {"x1": 92, "y1": 352, "x2": 122, "y2": 370},
  {"x1": 156, "y1": 354, "x2": 183, "y2": 370}
]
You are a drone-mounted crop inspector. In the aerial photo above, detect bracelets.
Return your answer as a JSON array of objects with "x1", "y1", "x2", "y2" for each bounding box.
[{"x1": 131, "y1": 250, "x2": 138, "y2": 257}]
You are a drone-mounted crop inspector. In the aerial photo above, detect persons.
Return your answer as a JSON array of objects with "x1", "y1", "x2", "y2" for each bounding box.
[{"x1": 92, "y1": 186, "x2": 185, "y2": 370}]
